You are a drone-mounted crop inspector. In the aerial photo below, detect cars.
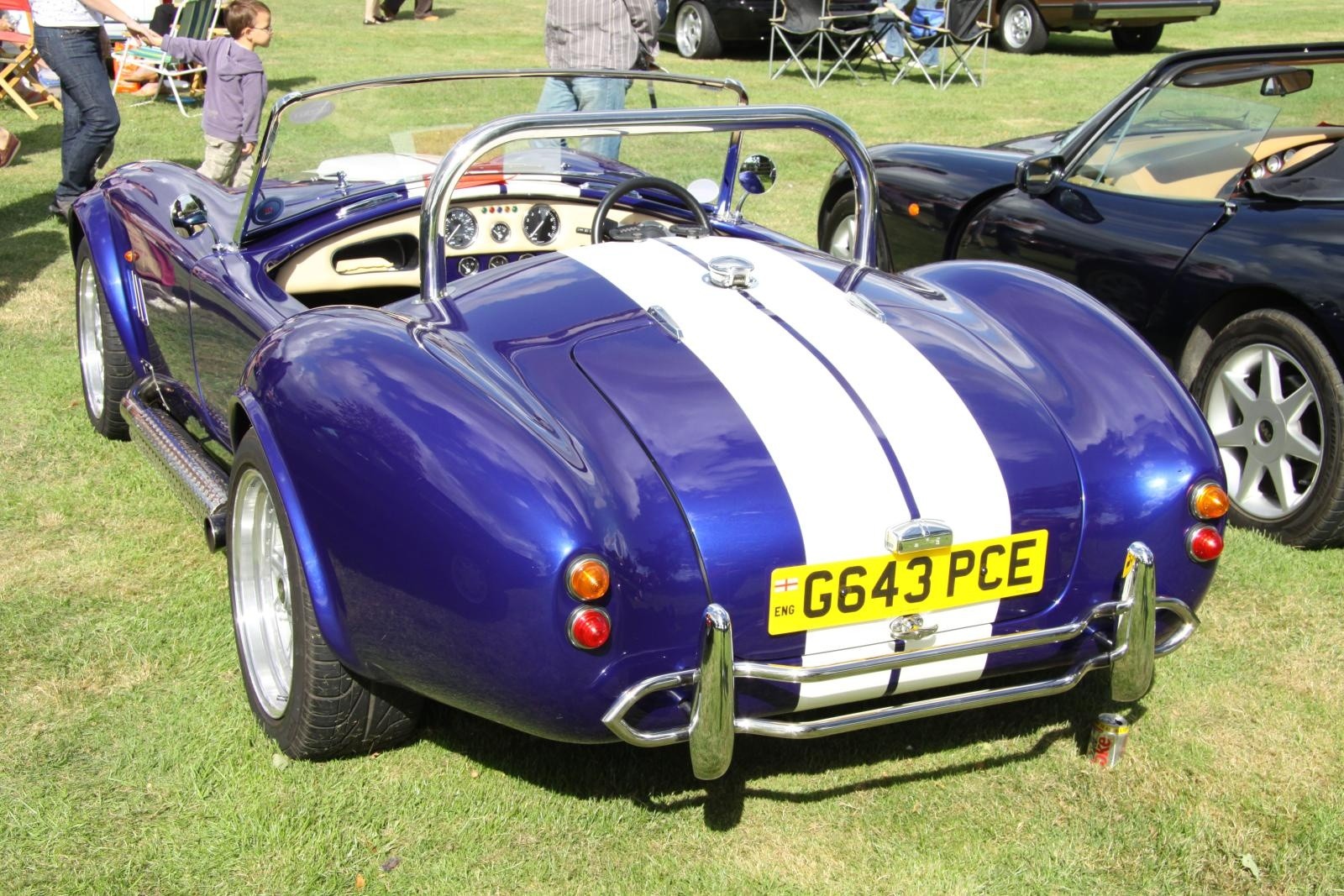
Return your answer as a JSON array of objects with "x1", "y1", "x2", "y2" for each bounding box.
[
  {"x1": 651, "y1": 0, "x2": 1223, "y2": 61},
  {"x1": 3, "y1": 1, "x2": 164, "y2": 81}
]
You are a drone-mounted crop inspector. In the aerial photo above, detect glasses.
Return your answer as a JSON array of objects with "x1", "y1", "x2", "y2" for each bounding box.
[{"x1": 251, "y1": 24, "x2": 272, "y2": 33}]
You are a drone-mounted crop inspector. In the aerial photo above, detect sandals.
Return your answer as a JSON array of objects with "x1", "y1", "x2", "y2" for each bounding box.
[
  {"x1": 374, "y1": 15, "x2": 387, "y2": 22},
  {"x1": 364, "y1": 19, "x2": 383, "y2": 25}
]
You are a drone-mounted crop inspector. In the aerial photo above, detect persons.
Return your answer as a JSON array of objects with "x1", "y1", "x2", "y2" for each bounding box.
[
  {"x1": 530, "y1": 0, "x2": 661, "y2": 162},
  {"x1": 0, "y1": 126, "x2": 21, "y2": 169},
  {"x1": 364, "y1": 0, "x2": 392, "y2": 25},
  {"x1": 869, "y1": 0, "x2": 941, "y2": 68},
  {"x1": 27, "y1": 0, "x2": 162, "y2": 223},
  {"x1": 379, "y1": 0, "x2": 440, "y2": 20},
  {"x1": 139, "y1": 0, "x2": 273, "y2": 186}
]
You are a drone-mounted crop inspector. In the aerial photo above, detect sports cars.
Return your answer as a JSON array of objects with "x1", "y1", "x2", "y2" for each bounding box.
[
  {"x1": 815, "y1": 38, "x2": 1344, "y2": 550},
  {"x1": 60, "y1": 68, "x2": 1232, "y2": 783}
]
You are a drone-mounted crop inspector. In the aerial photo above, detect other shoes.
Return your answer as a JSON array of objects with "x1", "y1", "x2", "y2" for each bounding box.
[
  {"x1": 0, "y1": 134, "x2": 21, "y2": 168},
  {"x1": 385, "y1": 14, "x2": 396, "y2": 21},
  {"x1": 414, "y1": 15, "x2": 439, "y2": 21},
  {"x1": 50, "y1": 198, "x2": 71, "y2": 215}
]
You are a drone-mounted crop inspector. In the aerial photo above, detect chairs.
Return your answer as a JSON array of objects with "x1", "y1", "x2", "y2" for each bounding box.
[
  {"x1": 767, "y1": 0, "x2": 991, "y2": 90},
  {"x1": 112, "y1": 0, "x2": 222, "y2": 119},
  {"x1": 0, "y1": 0, "x2": 62, "y2": 120}
]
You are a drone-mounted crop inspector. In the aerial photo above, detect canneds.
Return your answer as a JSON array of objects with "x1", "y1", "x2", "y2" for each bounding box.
[{"x1": 1087, "y1": 713, "x2": 1129, "y2": 771}]
